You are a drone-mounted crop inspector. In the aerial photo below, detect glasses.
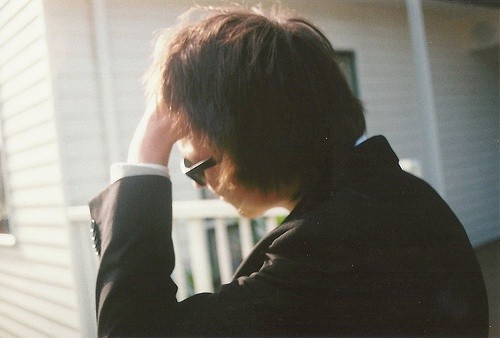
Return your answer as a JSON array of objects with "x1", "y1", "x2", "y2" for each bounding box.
[{"x1": 181, "y1": 158, "x2": 220, "y2": 187}]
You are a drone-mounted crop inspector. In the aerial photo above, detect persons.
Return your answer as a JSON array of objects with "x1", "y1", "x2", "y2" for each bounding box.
[{"x1": 84, "y1": 5, "x2": 494, "y2": 338}]
